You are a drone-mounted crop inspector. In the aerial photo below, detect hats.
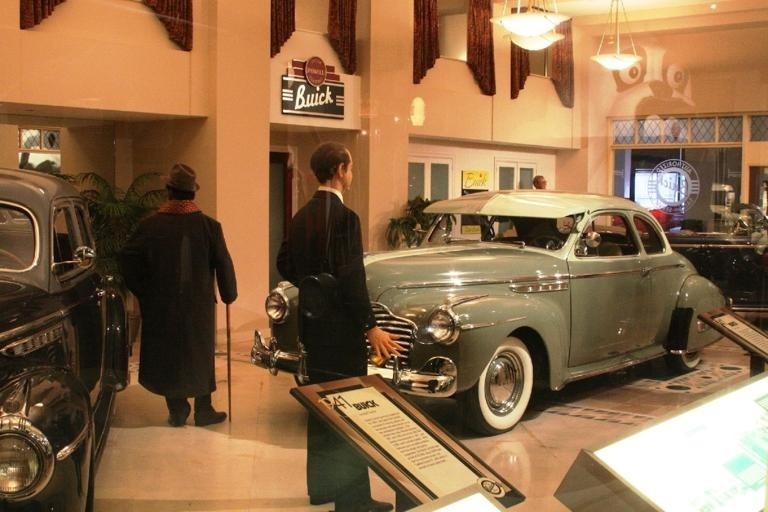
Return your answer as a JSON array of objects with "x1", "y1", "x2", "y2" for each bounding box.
[{"x1": 161, "y1": 165, "x2": 200, "y2": 192}]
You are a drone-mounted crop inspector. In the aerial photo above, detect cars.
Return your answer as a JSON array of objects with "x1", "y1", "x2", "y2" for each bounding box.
[
  {"x1": 0, "y1": 165, "x2": 136, "y2": 512},
  {"x1": 247, "y1": 186, "x2": 731, "y2": 435}
]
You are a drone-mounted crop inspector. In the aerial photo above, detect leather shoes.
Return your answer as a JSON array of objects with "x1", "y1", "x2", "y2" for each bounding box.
[
  {"x1": 168, "y1": 413, "x2": 226, "y2": 425},
  {"x1": 311, "y1": 496, "x2": 393, "y2": 512}
]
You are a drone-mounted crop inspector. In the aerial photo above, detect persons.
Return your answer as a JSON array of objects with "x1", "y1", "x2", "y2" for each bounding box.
[
  {"x1": 116, "y1": 161, "x2": 239, "y2": 427},
  {"x1": 276, "y1": 138, "x2": 408, "y2": 512},
  {"x1": 533, "y1": 175, "x2": 548, "y2": 189}
]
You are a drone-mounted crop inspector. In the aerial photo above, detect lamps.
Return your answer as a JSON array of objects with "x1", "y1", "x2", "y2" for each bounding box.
[
  {"x1": 488, "y1": 0, "x2": 573, "y2": 53},
  {"x1": 588, "y1": 0, "x2": 643, "y2": 73}
]
